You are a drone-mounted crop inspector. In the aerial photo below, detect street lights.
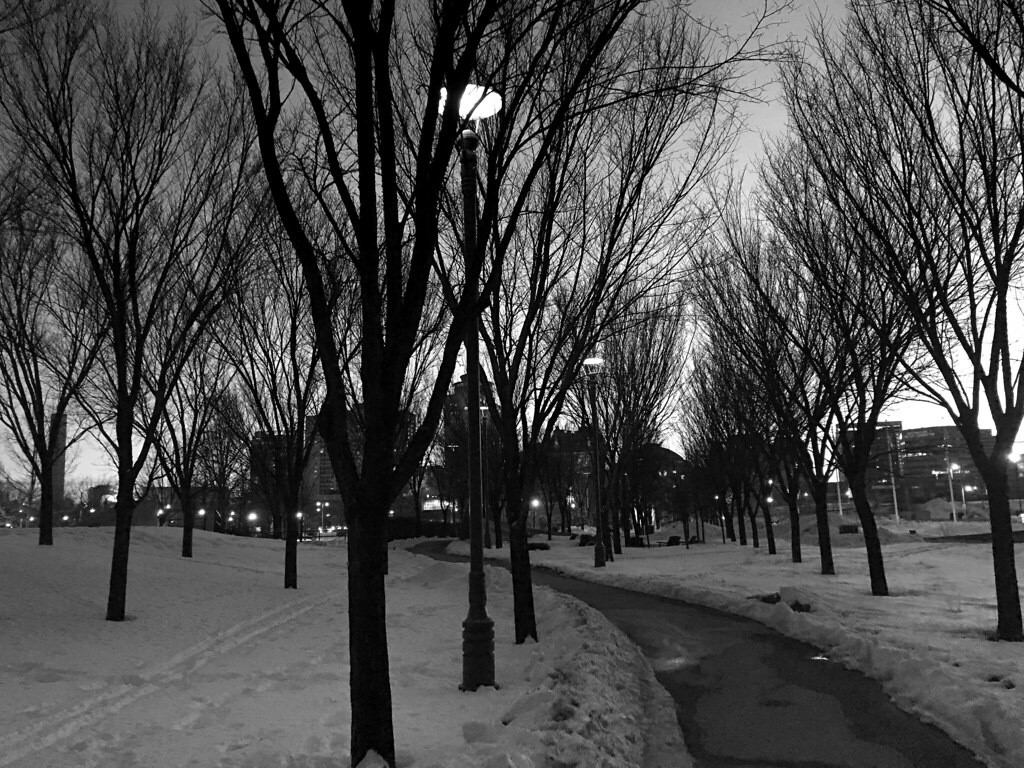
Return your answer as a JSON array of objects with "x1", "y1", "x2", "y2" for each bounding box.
[
  {"x1": 315, "y1": 502, "x2": 330, "y2": 528},
  {"x1": 581, "y1": 357, "x2": 608, "y2": 567},
  {"x1": 432, "y1": 83, "x2": 502, "y2": 693},
  {"x1": 297, "y1": 512, "x2": 304, "y2": 542},
  {"x1": 531, "y1": 500, "x2": 540, "y2": 530}
]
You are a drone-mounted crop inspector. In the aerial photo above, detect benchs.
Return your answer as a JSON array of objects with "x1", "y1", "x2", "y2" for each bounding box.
[
  {"x1": 682, "y1": 535, "x2": 698, "y2": 545},
  {"x1": 660, "y1": 535, "x2": 682, "y2": 547}
]
